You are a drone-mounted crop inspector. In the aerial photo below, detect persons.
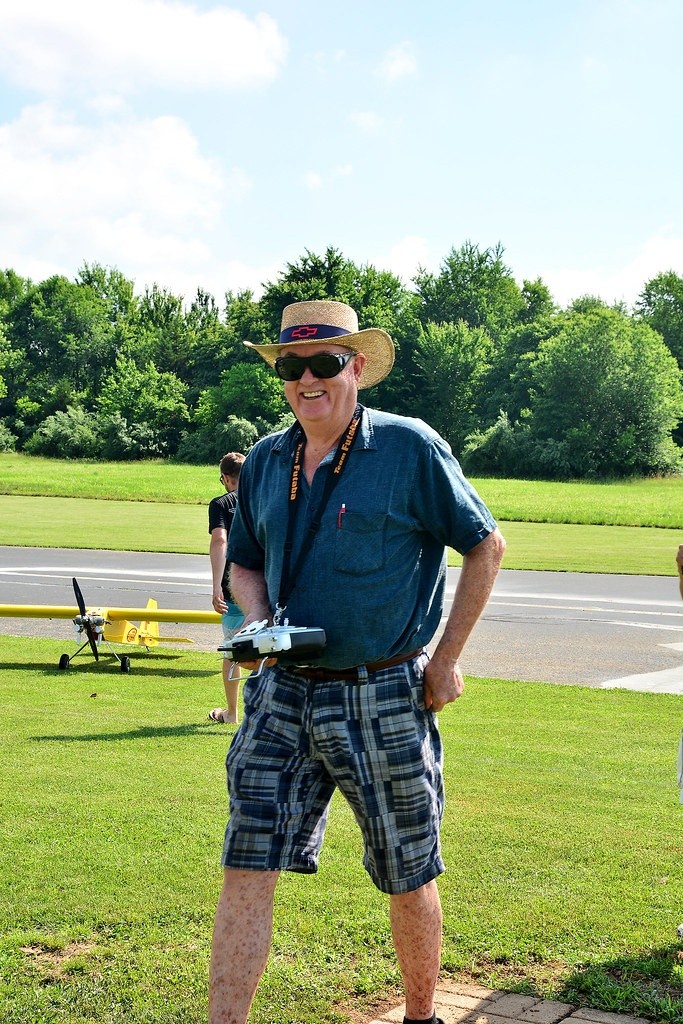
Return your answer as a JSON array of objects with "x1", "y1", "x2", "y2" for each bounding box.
[
  {"x1": 208, "y1": 451, "x2": 247, "y2": 722},
  {"x1": 207, "y1": 300, "x2": 506, "y2": 1024}
]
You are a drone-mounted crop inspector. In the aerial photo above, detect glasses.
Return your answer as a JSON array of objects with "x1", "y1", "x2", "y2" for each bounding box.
[{"x1": 275, "y1": 350, "x2": 355, "y2": 380}]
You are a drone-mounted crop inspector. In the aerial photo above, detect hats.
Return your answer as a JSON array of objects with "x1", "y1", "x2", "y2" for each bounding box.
[{"x1": 242, "y1": 301, "x2": 396, "y2": 390}]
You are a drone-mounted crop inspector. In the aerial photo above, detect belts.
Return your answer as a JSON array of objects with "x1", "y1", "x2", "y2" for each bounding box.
[{"x1": 301, "y1": 645, "x2": 427, "y2": 681}]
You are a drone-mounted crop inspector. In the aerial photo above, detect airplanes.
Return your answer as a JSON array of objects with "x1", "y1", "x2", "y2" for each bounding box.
[{"x1": 1, "y1": 579, "x2": 224, "y2": 674}]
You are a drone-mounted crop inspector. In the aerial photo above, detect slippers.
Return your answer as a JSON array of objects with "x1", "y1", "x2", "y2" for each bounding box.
[{"x1": 210, "y1": 707, "x2": 227, "y2": 724}]
